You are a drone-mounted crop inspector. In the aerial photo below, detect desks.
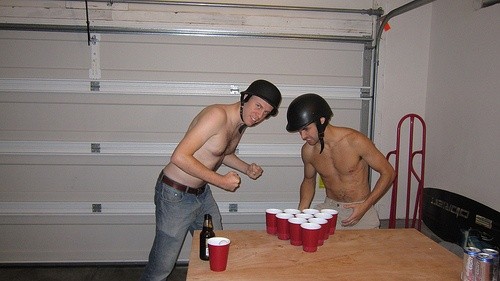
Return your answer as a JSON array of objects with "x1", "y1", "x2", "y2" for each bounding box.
[{"x1": 187, "y1": 228, "x2": 464, "y2": 281}]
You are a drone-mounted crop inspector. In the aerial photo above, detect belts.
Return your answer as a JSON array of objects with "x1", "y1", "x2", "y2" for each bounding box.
[{"x1": 159, "y1": 172, "x2": 207, "y2": 195}]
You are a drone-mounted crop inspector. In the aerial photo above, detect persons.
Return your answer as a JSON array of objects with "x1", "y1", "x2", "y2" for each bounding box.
[
  {"x1": 140, "y1": 79, "x2": 282, "y2": 281},
  {"x1": 286, "y1": 93, "x2": 396, "y2": 230}
]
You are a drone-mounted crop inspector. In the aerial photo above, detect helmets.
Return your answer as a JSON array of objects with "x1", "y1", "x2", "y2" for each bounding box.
[
  {"x1": 286, "y1": 94, "x2": 333, "y2": 133},
  {"x1": 241, "y1": 80, "x2": 282, "y2": 119}
]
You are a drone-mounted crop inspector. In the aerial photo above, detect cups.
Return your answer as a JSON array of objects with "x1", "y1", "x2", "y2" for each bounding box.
[
  {"x1": 207, "y1": 237, "x2": 231, "y2": 272},
  {"x1": 266, "y1": 208, "x2": 339, "y2": 252}
]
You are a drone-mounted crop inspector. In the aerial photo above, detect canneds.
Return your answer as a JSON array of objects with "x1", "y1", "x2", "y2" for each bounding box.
[{"x1": 461, "y1": 246, "x2": 498, "y2": 281}]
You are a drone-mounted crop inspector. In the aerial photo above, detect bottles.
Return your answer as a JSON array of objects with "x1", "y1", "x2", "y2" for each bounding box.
[{"x1": 199, "y1": 213, "x2": 216, "y2": 261}]
